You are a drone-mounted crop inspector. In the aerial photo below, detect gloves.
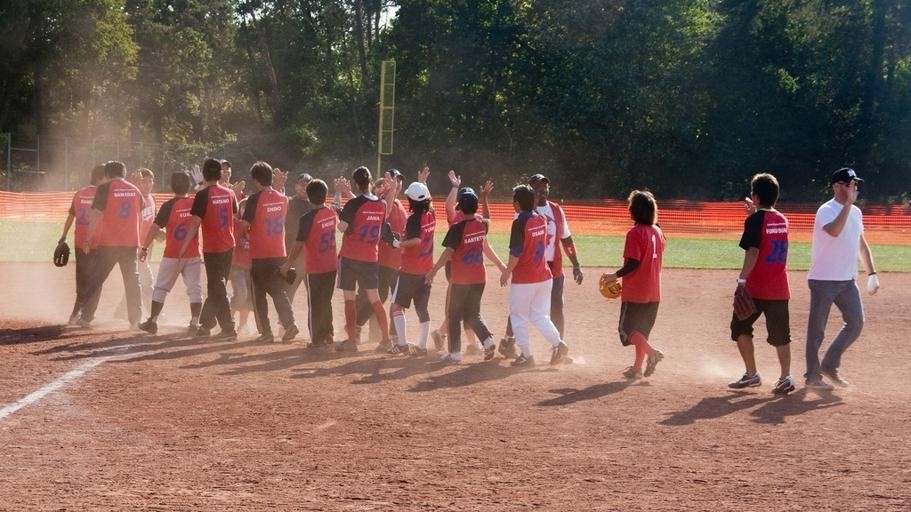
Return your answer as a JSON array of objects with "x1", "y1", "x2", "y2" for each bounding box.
[
  {"x1": 867, "y1": 270, "x2": 881, "y2": 297},
  {"x1": 188, "y1": 165, "x2": 206, "y2": 185}
]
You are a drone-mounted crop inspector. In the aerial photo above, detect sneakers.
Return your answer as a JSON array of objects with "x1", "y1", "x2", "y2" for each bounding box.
[
  {"x1": 410, "y1": 345, "x2": 430, "y2": 356},
  {"x1": 805, "y1": 376, "x2": 833, "y2": 391},
  {"x1": 336, "y1": 341, "x2": 358, "y2": 353},
  {"x1": 549, "y1": 342, "x2": 566, "y2": 366},
  {"x1": 307, "y1": 342, "x2": 334, "y2": 354},
  {"x1": 823, "y1": 367, "x2": 851, "y2": 388},
  {"x1": 68, "y1": 313, "x2": 81, "y2": 324},
  {"x1": 441, "y1": 352, "x2": 462, "y2": 363},
  {"x1": 772, "y1": 376, "x2": 796, "y2": 394},
  {"x1": 483, "y1": 337, "x2": 496, "y2": 361},
  {"x1": 282, "y1": 324, "x2": 299, "y2": 341},
  {"x1": 192, "y1": 330, "x2": 213, "y2": 341},
  {"x1": 212, "y1": 332, "x2": 238, "y2": 341},
  {"x1": 374, "y1": 339, "x2": 394, "y2": 353},
  {"x1": 254, "y1": 335, "x2": 275, "y2": 343},
  {"x1": 498, "y1": 338, "x2": 520, "y2": 359},
  {"x1": 387, "y1": 344, "x2": 409, "y2": 355},
  {"x1": 137, "y1": 322, "x2": 160, "y2": 333},
  {"x1": 645, "y1": 348, "x2": 665, "y2": 378},
  {"x1": 430, "y1": 328, "x2": 447, "y2": 352},
  {"x1": 621, "y1": 367, "x2": 644, "y2": 378},
  {"x1": 77, "y1": 319, "x2": 96, "y2": 328},
  {"x1": 464, "y1": 345, "x2": 483, "y2": 356},
  {"x1": 186, "y1": 325, "x2": 199, "y2": 336},
  {"x1": 510, "y1": 354, "x2": 535, "y2": 368},
  {"x1": 564, "y1": 358, "x2": 574, "y2": 364},
  {"x1": 728, "y1": 371, "x2": 762, "y2": 389}
]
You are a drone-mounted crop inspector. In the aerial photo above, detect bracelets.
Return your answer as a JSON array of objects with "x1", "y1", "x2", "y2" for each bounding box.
[
  {"x1": 868, "y1": 271, "x2": 877, "y2": 276},
  {"x1": 737, "y1": 278, "x2": 747, "y2": 283}
]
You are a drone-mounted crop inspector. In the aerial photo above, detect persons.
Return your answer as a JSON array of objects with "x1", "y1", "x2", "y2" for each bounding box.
[
  {"x1": 728, "y1": 173, "x2": 795, "y2": 394},
  {"x1": 802, "y1": 166, "x2": 881, "y2": 390},
  {"x1": 603, "y1": 192, "x2": 665, "y2": 379},
  {"x1": 58, "y1": 158, "x2": 583, "y2": 366}
]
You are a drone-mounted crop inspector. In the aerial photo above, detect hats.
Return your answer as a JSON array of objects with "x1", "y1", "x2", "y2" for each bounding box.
[
  {"x1": 403, "y1": 182, "x2": 433, "y2": 201},
  {"x1": 530, "y1": 172, "x2": 552, "y2": 188},
  {"x1": 388, "y1": 169, "x2": 406, "y2": 180},
  {"x1": 598, "y1": 273, "x2": 622, "y2": 300},
  {"x1": 458, "y1": 187, "x2": 480, "y2": 199},
  {"x1": 220, "y1": 159, "x2": 232, "y2": 169},
  {"x1": 829, "y1": 167, "x2": 865, "y2": 187}
]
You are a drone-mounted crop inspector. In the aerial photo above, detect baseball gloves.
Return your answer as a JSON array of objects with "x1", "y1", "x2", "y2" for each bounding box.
[
  {"x1": 54, "y1": 242, "x2": 70, "y2": 269},
  {"x1": 281, "y1": 267, "x2": 297, "y2": 287},
  {"x1": 734, "y1": 279, "x2": 757, "y2": 322}
]
